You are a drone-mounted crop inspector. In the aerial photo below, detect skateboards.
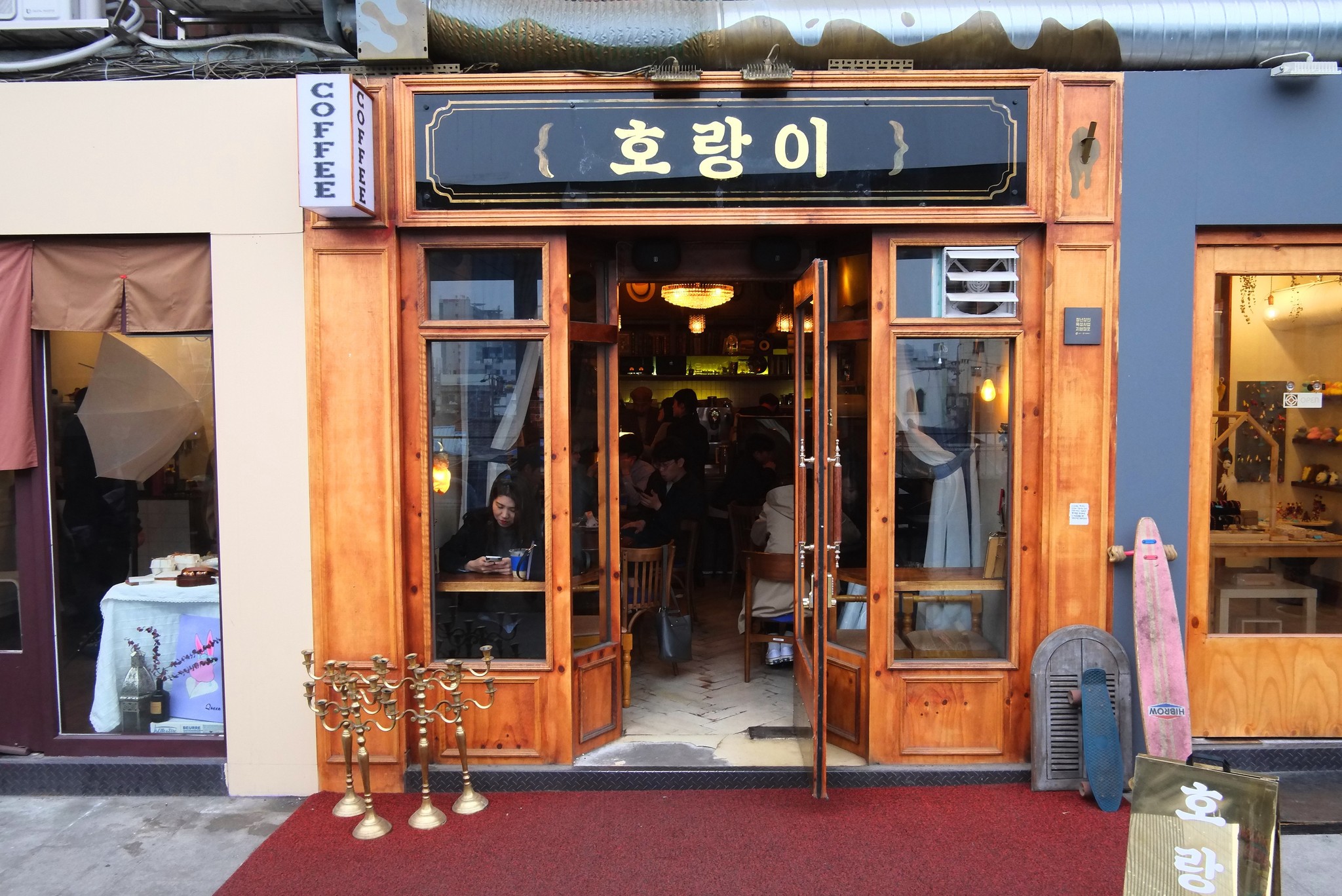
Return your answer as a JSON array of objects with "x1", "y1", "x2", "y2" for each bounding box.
[
  {"x1": 1106, "y1": 517, "x2": 1196, "y2": 790},
  {"x1": 1065, "y1": 668, "x2": 1127, "y2": 812}
]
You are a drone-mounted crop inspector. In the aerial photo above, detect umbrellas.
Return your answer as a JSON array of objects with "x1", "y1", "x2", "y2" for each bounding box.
[{"x1": 73, "y1": 333, "x2": 212, "y2": 486}]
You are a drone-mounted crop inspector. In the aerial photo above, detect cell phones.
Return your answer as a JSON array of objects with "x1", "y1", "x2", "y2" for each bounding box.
[
  {"x1": 485, "y1": 556, "x2": 502, "y2": 564},
  {"x1": 633, "y1": 486, "x2": 645, "y2": 498}
]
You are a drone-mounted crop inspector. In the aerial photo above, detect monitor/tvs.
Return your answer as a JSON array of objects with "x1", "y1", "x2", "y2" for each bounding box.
[{"x1": 656, "y1": 353, "x2": 687, "y2": 375}]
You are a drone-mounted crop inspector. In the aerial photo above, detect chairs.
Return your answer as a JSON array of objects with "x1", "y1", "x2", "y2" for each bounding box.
[
  {"x1": 836, "y1": 593, "x2": 912, "y2": 661},
  {"x1": 570, "y1": 501, "x2": 814, "y2": 710},
  {"x1": 900, "y1": 594, "x2": 1000, "y2": 662}
]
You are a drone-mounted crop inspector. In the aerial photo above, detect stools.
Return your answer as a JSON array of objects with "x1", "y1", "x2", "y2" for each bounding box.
[{"x1": 1236, "y1": 615, "x2": 1282, "y2": 635}]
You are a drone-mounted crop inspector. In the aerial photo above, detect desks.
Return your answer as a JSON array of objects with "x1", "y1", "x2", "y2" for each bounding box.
[
  {"x1": 572, "y1": 517, "x2": 634, "y2": 551},
  {"x1": 1277, "y1": 519, "x2": 1332, "y2": 526},
  {"x1": 435, "y1": 571, "x2": 600, "y2": 592},
  {"x1": 89, "y1": 558, "x2": 226, "y2": 739},
  {"x1": 836, "y1": 566, "x2": 1007, "y2": 591},
  {"x1": 1208, "y1": 539, "x2": 1342, "y2": 619}
]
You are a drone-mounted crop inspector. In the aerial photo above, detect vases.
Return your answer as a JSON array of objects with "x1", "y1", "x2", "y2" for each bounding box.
[{"x1": 150, "y1": 680, "x2": 170, "y2": 723}]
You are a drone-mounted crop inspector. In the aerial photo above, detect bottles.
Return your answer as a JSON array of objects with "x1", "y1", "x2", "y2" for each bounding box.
[
  {"x1": 150, "y1": 678, "x2": 170, "y2": 723},
  {"x1": 719, "y1": 446, "x2": 729, "y2": 462},
  {"x1": 166, "y1": 464, "x2": 176, "y2": 493}
]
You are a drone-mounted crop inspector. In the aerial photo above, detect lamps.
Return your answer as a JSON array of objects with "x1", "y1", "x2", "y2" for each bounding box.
[
  {"x1": 433, "y1": 440, "x2": 451, "y2": 495},
  {"x1": 1258, "y1": 51, "x2": 1342, "y2": 76},
  {"x1": 804, "y1": 311, "x2": 813, "y2": 333},
  {"x1": 776, "y1": 303, "x2": 793, "y2": 332},
  {"x1": 689, "y1": 315, "x2": 706, "y2": 334},
  {"x1": 661, "y1": 282, "x2": 733, "y2": 309}
]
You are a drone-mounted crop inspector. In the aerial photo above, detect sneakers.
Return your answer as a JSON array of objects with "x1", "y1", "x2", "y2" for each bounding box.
[
  {"x1": 765, "y1": 647, "x2": 781, "y2": 664},
  {"x1": 780, "y1": 647, "x2": 794, "y2": 662}
]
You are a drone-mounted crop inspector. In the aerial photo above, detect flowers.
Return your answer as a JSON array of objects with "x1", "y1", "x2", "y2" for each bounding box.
[{"x1": 124, "y1": 626, "x2": 222, "y2": 680}]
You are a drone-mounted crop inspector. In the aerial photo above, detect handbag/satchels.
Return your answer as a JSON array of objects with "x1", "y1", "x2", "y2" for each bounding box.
[
  {"x1": 525, "y1": 540, "x2": 546, "y2": 581},
  {"x1": 660, "y1": 612, "x2": 693, "y2": 662}
]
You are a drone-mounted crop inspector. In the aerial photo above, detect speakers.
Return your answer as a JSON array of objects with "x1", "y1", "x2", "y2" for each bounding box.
[
  {"x1": 751, "y1": 234, "x2": 801, "y2": 271},
  {"x1": 812, "y1": 239, "x2": 833, "y2": 267},
  {"x1": 754, "y1": 333, "x2": 775, "y2": 356},
  {"x1": 631, "y1": 234, "x2": 682, "y2": 273}
]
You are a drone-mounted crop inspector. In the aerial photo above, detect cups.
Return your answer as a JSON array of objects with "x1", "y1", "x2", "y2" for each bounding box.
[
  {"x1": 509, "y1": 548, "x2": 529, "y2": 578},
  {"x1": 789, "y1": 393, "x2": 794, "y2": 405},
  {"x1": 729, "y1": 361, "x2": 738, "y2": 375},
  {"x1": 780, "y1": 395, "x2": 789, "y2": 405},
  {"x1": 708, "y1": 396, "x2": 717, "y2": 407}
]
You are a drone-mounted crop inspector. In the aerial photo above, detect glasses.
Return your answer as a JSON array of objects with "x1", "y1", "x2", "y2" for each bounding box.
[{"x1": 654, "y1": 458, "x2": 681, "y2": 472}]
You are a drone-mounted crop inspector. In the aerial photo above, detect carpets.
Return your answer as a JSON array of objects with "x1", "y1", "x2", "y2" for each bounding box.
[{"x1": 212, "y1": 782, "x2": 1132, "y2": 896}]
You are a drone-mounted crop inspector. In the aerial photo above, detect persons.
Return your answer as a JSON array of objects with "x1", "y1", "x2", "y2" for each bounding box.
[
  {"x1": 737, "y1": 461, "x2": 866, "y2": 667},
  {"x1": 713, "y1": 394, "x2": 780, "y2": 577},
  {"x1": 55, "y1": 387, "x2": 145, "y2": 657},
  {"x1": 441, "y1": 469, "x2": 546, "y2": 658},
  {"x1": 519, "y1": 386, "x2": 711, "y2": 596}
]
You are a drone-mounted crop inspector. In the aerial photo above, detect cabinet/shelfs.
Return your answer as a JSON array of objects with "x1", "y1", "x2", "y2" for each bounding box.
[{"x1": 1291, "y1": 394, "x2": 1342, "y2": 493}]
